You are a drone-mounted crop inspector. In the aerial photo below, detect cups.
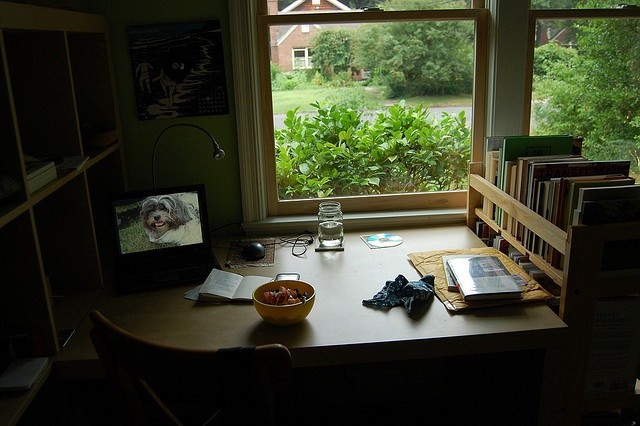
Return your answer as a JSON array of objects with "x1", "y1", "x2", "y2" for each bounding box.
[{"x1": 317, "y1": 201, "x2": 344, "y2": 247}]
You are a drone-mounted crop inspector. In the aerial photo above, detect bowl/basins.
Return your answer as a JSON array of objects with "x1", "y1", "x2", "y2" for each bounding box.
[{"x1": 252, "y1": 280, "x2": 316, "y2": 327}]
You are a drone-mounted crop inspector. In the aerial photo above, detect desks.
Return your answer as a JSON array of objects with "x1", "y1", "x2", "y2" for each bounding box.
[{"x1": 92, "y1": 223, "x2": 568, "y2": 425}]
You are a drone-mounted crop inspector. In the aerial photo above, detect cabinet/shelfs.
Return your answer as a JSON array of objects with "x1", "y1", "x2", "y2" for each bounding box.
[{"x1": 468, "y1": 136, "x2": 640, "y2": 426}]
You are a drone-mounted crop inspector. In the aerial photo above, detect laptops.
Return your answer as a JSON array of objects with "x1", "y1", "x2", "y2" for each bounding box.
[{"x1": 101, "y1": 183, "x2": 222, "y2": 296}]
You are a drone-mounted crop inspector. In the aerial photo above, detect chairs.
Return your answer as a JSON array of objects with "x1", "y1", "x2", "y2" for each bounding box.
[{"x1": 87, "y1": 310, "x2": 293, "y2": 426}]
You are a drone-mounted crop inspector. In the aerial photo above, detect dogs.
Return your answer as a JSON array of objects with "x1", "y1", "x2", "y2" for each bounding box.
[{"x1": 139, "y1": 194, "x2": 203, "y2": 246}]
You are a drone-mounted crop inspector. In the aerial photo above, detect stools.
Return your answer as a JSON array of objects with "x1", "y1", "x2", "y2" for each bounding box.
[{"x1": 0, "y1": 1, "x2": 127, "y2": 425}]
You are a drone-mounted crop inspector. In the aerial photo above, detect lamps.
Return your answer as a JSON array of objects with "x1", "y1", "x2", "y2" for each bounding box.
[{"x1": 209, "y1": 144, "x2": 228, "y2": 161}]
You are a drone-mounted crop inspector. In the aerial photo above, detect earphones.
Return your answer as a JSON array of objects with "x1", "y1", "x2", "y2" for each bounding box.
[{"x1": 309, "y1": 237, "x2": 314, "y2": 244}]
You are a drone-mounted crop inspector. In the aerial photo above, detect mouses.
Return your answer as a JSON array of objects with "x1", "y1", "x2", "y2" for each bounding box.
[{"x1": 244, "y1": 241, "x2": 265, "y2": 260}]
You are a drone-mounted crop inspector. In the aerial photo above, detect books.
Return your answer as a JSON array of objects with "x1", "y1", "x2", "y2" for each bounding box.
[
  {"x1": 484, "y1": 136, "x2": 640, "y2": 271},
  {"x1": 185, "y1": 266, "x2": 274, "y2": 303},
  {"x1": 25, "y1": 160, "x2": 59, "y2": 196},
  {"x1": 443, "y1": 254, "x2": 524, "y2": 302},
  {"x1": 475, "y1": 221, "x2": 639, "y2": 392},
  {"x1": 0, "y1": 357, "x2": 49, "y2": 392}
]
